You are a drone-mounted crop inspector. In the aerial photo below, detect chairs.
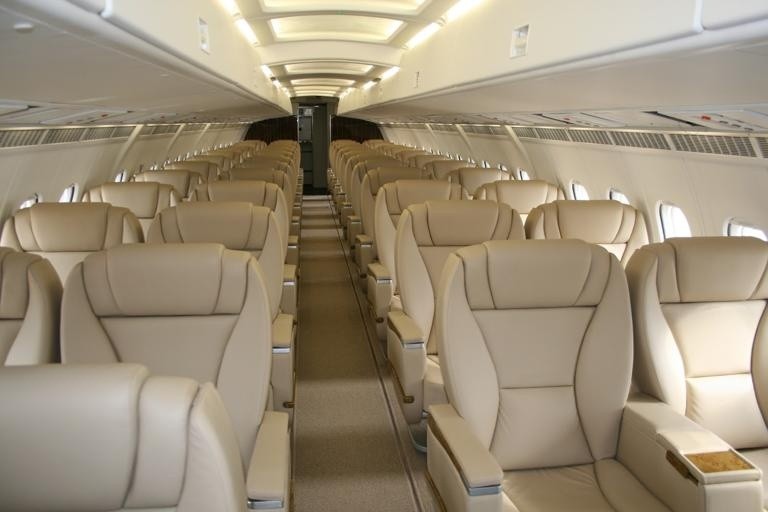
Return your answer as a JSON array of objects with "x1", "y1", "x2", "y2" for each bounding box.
[
  {"x1": 1, "y1": 139, "x2": 305, "y2": 511},
  {"x1": 325, "y1": 140, "x2": 768, "y2": 510}
]
